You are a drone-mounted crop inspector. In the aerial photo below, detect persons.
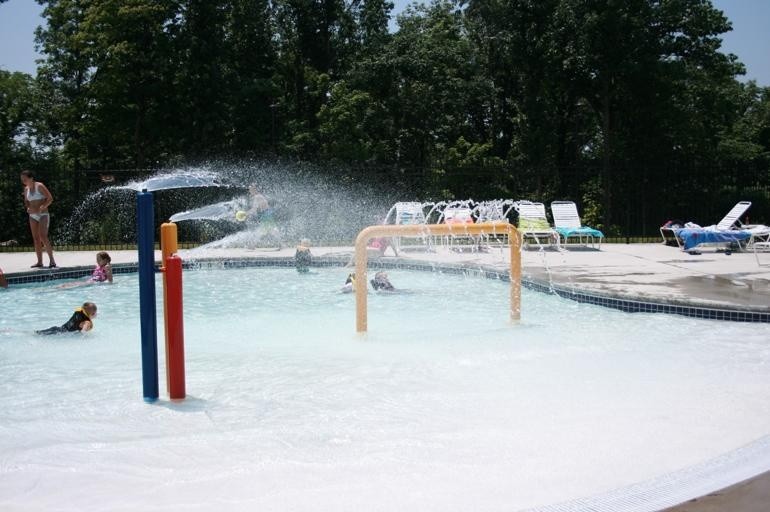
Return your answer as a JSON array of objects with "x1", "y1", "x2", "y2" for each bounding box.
[
  {"x1": 246, "y1": 183, "x2": 286, "y2": 251},
  {"x1": 20, "y1": 169, "x2": 56, "y2": 268},
  {"x1": 345, "y1": 237, "x2": 398, "y2": 269},
  {"x1": 2, "y1": 300, "x2": 97, "y2": 336},
  {"x1": 33, "y1": 252, "x2": 113, "y2": 292},
  {"x1": 295, "y1": 238, "x2": 310, "y2": 272},
  {"x1": 339, "y1": 271, "x2": 355, "y2": 293},
  {"x1": 372, "y1": 270, "x2": 401, "y2": 293}
]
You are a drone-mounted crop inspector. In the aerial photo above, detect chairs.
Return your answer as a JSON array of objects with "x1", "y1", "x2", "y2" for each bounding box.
[
  {"x1": 659, "y1": 200, "x2": 770, "y2": 252},
  {"x1": 384, "y1": 200, "x2": 604, "y2": 253}
]
